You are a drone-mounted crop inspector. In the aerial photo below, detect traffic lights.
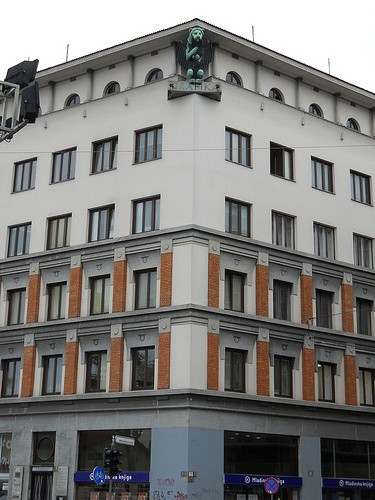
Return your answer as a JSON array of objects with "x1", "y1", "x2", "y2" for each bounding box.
[{"x1": 102, "y1": 448, "x2": 122, "y2": 479}]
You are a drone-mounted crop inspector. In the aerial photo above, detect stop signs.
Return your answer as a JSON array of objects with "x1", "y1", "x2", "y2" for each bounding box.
[{"x1": 265, "y1": 478, "x2": 278, "y2": 494}]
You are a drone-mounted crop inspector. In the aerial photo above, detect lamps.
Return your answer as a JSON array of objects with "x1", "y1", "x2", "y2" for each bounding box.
[
  {"x1": 2, "y1": 58, "x2": 39, "y2": 94},
  {"x1": 19, "y1": 82, "x2": 41, "y2": 124}
]
